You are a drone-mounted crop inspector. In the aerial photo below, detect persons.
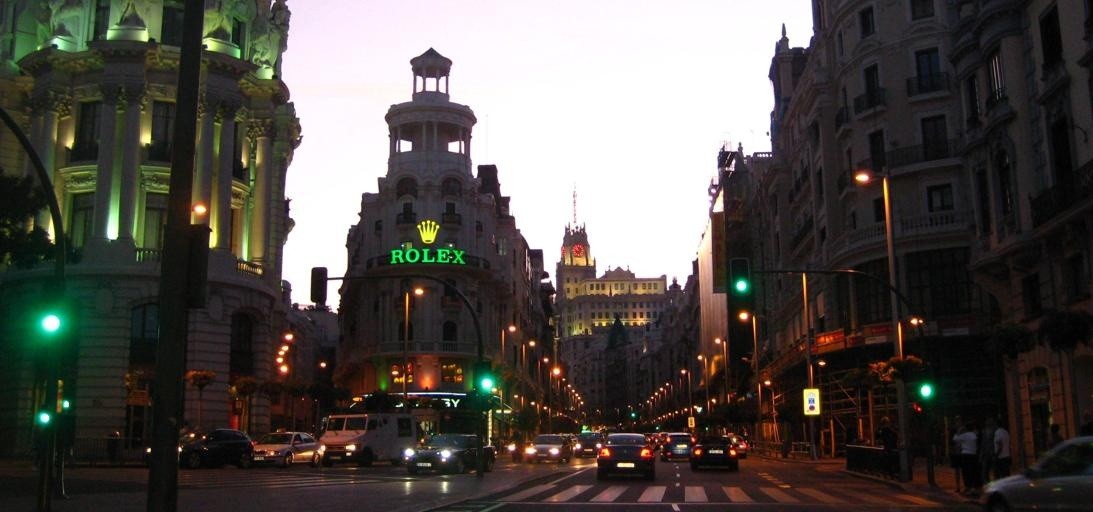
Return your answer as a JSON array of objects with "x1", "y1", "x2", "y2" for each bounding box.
[
  {"x1": 106, "y1": 431, "x2": 122, "y2": 460},
  {"x1": 1046, "y1": 423, "x2": 1064, "y2": 449},
  {"x1": 30, "y1": 434, "x2": 40, "y2": 473},
  {"x1": 948, "y1": 415, "x2": 1011, "y2": 497},
  {"x1": 874, "y1": 416, "x2": 898, "y2": 439},
  {"x1": 510, "y1": 430, "x2": 524, "y2": 451},
  {"x1": 1079, "y1": 407, "x2": 1093, "y2": 437}
]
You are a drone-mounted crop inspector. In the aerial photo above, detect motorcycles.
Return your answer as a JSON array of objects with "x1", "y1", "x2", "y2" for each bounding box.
[{"x1": 509, "y1": 442, "x2": 524, "y2": 462}]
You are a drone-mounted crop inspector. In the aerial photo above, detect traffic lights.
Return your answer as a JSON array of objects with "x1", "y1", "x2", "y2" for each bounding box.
[
  {"x1": 39, "y1": 407, "x2": 54, "y2": 428},
  {"x1": 912, "y1": 401, "x2": 924, "y2": 416},
  {"x1": 731, "y1": 257, "x2": 754, "y2": 298},
  {"x1": 62, "y1": 399, "x2": 71, "y2": 411},
  {"x1": 916, "y1": 380, "x2": 938, "y2": 403},
  {"x1": 474, "y1": 360, "x2": 492, "y2": 393},
  {"x1": 39, "y1": 311, "x2": 64, "y2": 339}
]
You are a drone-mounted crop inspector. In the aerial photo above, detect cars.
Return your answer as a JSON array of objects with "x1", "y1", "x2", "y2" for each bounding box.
[
  {"x1": 250, "y1": 429, "x2": 326, "y2": 469},
  {"x1": 404, "y1": 434, "x2": 497, "y2": 478},
  {"x1": 981, "y1": 435, "x2": 1093, "y2": 511},
  {"x1": 143, "y1": 428, "x2": 254, "y2": 468},
  {"x1": 595, "y1": 433, "x2": 657, "y2": 481},
  {"x1": 651, "y1": 432, "x2": 752, "y2": 473},
  {"x1": 525, "y1": 432, "x2": 604, "y2": 466}
]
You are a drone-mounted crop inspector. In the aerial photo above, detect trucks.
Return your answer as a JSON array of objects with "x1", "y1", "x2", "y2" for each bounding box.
[{"x1": 320, "y1": 412, "x2": 417, "y2": 467}]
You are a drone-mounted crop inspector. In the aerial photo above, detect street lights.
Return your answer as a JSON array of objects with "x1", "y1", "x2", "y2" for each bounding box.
[
  {"x1": 697, "y1": 353, "x2": 712, "y2": 432},
  {"x1": 714, "y1": 336, "x2": 732, "y2": 431},
  {"x1": 537, "y1": 356, "x2": 550, "y2": 433},
  {"x1": 521, "y1": 338, "x2": 536, "y2": 432},
  {"x1": 855, "y1": 168, "x2": 913, "y2": 484},
  {"x1": 738, "y1": 310, "x2": 763, "y2": 454},
  {"x1": 403, "y1": 288, "x2": 425, "y2": 409},
  {"x1": 614, "y1": 368, "x2": 696, "y2": 433},
  {"x1": 501, "y1": 325, "x2": 516, "y2": 442},
  {"x1": 548, "y1": 366, "x2": 587, "y2": 433}
]
